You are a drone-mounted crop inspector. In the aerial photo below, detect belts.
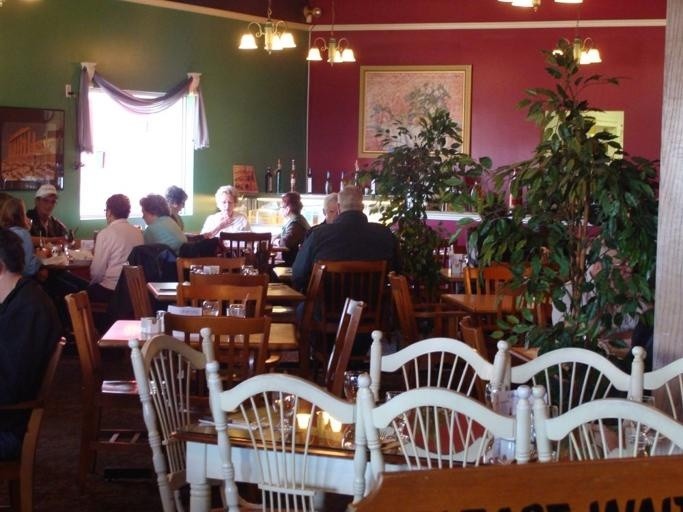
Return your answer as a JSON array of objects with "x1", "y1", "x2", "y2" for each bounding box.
[
  {"x1": 340, "y1": 371, "x2": 361, "y2": 401},
  {"x1": 486, "y1": 381, "x2": 504, "y2": 411},
  {"x1": 629, "y1": 394, "x2": 656, "y2": 445},
  {"x1": 386, "y1": 389, "x2": 411, "y2": 439},
  {"x1": 271, "y1": 390, "x2": 297, "y2": 430}
]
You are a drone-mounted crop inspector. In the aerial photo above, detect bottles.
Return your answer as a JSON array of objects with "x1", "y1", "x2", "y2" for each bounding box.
[{"x1": 34, "y1": 184, "x2": 57, "y2": 200}]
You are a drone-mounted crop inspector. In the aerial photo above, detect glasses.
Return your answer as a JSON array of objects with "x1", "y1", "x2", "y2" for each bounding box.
[
  {"x1": 238, "y1": 0, "x2": 296, "y2": 55},
  {"x1": 553, "y1": 5, "x2": 603, "y2": 65},
  {"x1": 306, "y1": 0, "x2": 356, "y2": 65},
  {"x1": 497, "y1": 0, "x2": 583, "y2": 15}
]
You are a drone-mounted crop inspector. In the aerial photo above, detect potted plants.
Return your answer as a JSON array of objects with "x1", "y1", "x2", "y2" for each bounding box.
[
  {"x1": 357, "y1": 63, "x2": 473, "y2": 160},
  {"x1": 0, "y1": 105, "x2": 65, "y2": 191}
]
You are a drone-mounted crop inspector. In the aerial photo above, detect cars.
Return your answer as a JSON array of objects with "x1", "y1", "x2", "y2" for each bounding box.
[
  {"x1": 241, "y1": 265, "x2": 259, "y2": 275},
  {"x1": 189, "y1": 265, "x2": 203, "y2": 274},
  {"x1": 202, "y1": 300, "x2": 245, "y2": 318},
  {"x1": 140, "y1": 310, "x2": 166, "y2": 338}
]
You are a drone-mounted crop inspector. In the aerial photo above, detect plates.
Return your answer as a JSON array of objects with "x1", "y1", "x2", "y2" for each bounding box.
[{"x1": 238, "y1": 198, "x2": 327, "y2": 233}]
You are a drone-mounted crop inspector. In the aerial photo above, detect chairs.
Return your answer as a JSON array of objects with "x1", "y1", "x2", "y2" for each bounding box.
[{"x1": 0, "y1": 233, "x2": 683, "y2": 512}]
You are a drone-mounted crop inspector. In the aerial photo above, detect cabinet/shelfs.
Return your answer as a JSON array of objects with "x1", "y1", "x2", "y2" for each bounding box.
[
  {"x1": 238, "y1": 198, "x2": 327, "y2": 233},
  {"x1": 265, "y1": 158, "x2": 360, "y2": 194},
  {"x1": 446, "y1": 167, "x2": 523, "y2": 214}
]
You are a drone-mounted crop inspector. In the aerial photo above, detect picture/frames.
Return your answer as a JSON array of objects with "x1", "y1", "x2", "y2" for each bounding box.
[
  {"x1": 357, "y1": 63, "x2": 473, "y2": 160},
  {"x1": 0, "y1": 105, "x2": 65, "y2": 191}
]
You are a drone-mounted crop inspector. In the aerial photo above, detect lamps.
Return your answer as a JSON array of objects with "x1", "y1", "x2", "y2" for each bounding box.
[
  {"x1": 238, "y1": 0, "x2": 296, "y2": 55},
  {"x1": 553, "y1": 5, "x2": 603, "y2": 65},
  {"x1": 497, "y1": 0, "x2": 583, "y2": 15},
  {"x1": 306, "y1": 0, "x2": 356, "y2": 65}
]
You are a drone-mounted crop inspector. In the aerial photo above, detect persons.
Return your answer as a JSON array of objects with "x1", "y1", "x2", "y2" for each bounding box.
[
  {"x1": 1, "y1": 191, "x2": 42, "y2": 276},
  {"x1": 200, "y1": 185, "x2": 252, "y2": 247},
  {"x1": 88, "y1": 193, "x2": 144, "y2": 302},
  {"x1": 166, "y1": 184, "x2": 234, "y2": 244},
  {"x1": 291, "y1": 184, "x2": 403, "y2": 356},
  {"x1": 271, "y1": 192, "x2": 311, "y2": 267},
  {"x1": 26, "y1": 184, "x2": 90, "y2": 328},
  {"x1": 0, "y1": 225, "x2": 64, "y2": 461},
  {"x1": 140, "y1": 194, "x2": 187, "y2": 257},
  {"x1": 285, "y1": 192, "x2": 338, "y2": 267}
]
